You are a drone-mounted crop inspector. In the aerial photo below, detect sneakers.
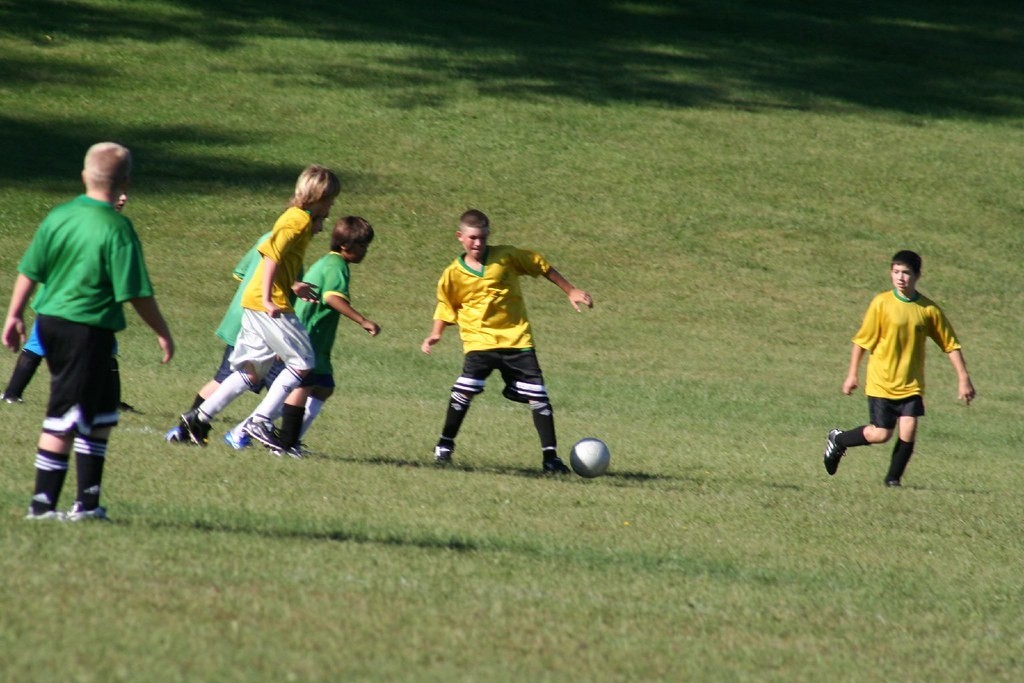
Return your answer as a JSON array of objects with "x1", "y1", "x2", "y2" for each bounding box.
[
  {"x1": 884, "y1": 479, "x2": 901, "y2": 487},
  {"x1": 181, "y1": 410, "x2": 212, "y2": 448},
  {"x1": 224, "y1": 432, "x2": 253, "y2": 449},
  {"x1": 242, "y1": 417, "x2": 282, "y2": 451},
  {"x1": 543, "y1": 455, "x2": 569, "y2": 474},
  {"x1": 166, "y1": 427, "x2": 181, "y2": 442},
  {"x1": 27, "y1": 509, "x2": 64, "y2": 520},
  {"x1": 823, "y1": 429, "x2": 847, "y2": 475},
  {"x1": 298, "y1": 444, "x2": 311, "y2": 456},
  {"x1": 66, "y1": 504, "x2": 112, "y2": 524},
  {"x1": 434, "y1": 446, "x2": 451, "y2": 468},
  {"x1": 269, "y1": 446, "x2": 301, "y2": 458}
]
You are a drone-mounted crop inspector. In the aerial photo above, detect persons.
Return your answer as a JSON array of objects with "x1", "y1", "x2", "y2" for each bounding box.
[
  {"x1": 0, "y1": 188, "x2": 134, "y2": 412},
  {"x1": 1, "y1": 142, "x2": 175, "y2": 521},
  {"x1": 179, "y1": 164, "x2": 340, "y2": 457},
  {"x1": 165, "y1": 213, "x2": 327, "y2": 456},
  {"x1": 823, "y1": 249, "x2": 976, "y2": 488},
  {"x1": 420, "y1": 211, "x2": 593, "y2": 475},
  {"x1": 226, "y1": 213, "x2": 381, "y2": 450}
]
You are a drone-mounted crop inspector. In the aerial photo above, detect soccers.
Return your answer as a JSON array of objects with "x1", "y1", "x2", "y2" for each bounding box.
[{"x1": 570, "y1": 438, "x2": 610, "y2": 478}]
